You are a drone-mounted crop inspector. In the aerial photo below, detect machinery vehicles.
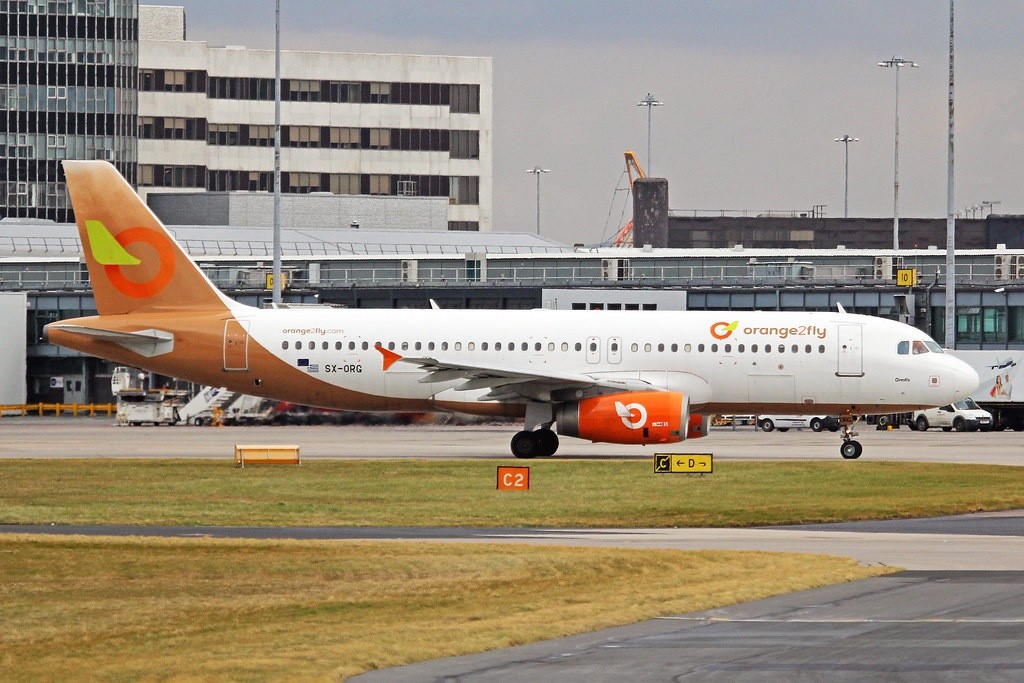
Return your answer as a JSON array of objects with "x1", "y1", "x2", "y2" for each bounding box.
[{"x1": 113, "y1": 364, "x2": 505, "y2": 429}]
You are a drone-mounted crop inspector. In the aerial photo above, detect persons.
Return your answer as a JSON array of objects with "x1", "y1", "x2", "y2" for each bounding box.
[{"x1": 989, "y1": 374, "x2": 1013, "y2": 399}]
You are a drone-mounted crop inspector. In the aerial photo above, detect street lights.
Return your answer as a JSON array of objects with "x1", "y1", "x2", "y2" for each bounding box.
[
  {"x1": 878, "y1": 53, "x2": 918, "y2": 250},
  {"x1": 834, "y1": 136, "x2": 861, "y2": 219},
  {"x1": 638, "y1": 93, "x2": 665, "y2": 182},
  {"x1": 525, "y1": 164, "x2": 552, "y2": 235}
]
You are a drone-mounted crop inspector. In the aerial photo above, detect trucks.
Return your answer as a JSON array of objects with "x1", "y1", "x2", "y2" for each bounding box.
[
  {"x1": 752, "y1": 409, "x2": 844, "y2": 434},
  {"x1": 909, "y1": 390, "x2": 993, "y2": 433}
]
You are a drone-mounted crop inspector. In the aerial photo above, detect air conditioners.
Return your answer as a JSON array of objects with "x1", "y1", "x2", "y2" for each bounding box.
[
  {"x1": 601, "y1": 270, "x2": 618, "y2": 281},
  {"x1": 993, "y1": 267, "x2": 1011, "y2": 281},
  {"x1": 400, "y1": 260, "x2": 418, "y2": 272},
  {"x1": 50, "y1": 375, "x2": 65, "y2": 388},
  {"x1": 873, "y1": 268, "x2": 892, "y2": 281},
  {"x1": 874, "y1": 256, "x2": 893, "y2": 269},
  {"x1": 401, "y1": 271, "x2": 418, "y2": 283},
  {"x1": 601, "y1": 259, "x2": 618, "y2": 270},
  {"x1": 994, "y1": 255, "x2": 1011, "y2": 267},
  {"x1": 1010, "y1": 255, "x2": 1024, "y2": 268},
  {"x1": 1010, "y1": 267, "x2": 1024, "y2": 280}
]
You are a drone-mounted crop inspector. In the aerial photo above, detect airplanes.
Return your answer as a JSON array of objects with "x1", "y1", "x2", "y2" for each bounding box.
[{"x1": 39, "y1": 156, "x2": 979, "y2": 463}]
